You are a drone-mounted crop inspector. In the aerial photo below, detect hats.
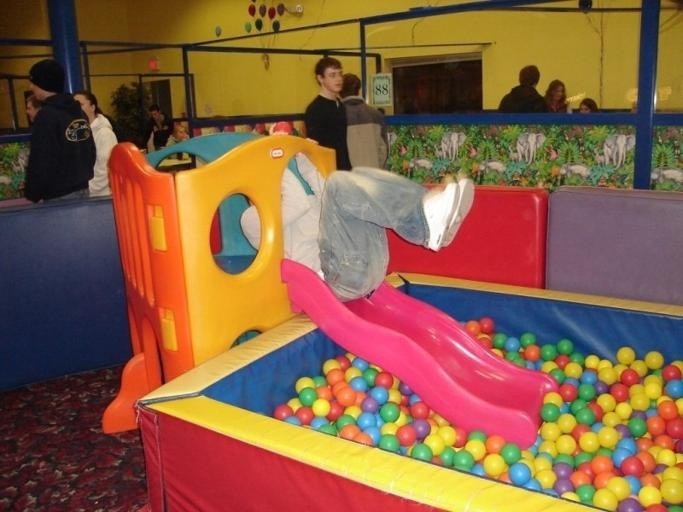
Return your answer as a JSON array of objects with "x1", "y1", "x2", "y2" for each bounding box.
[
  {"x1": 28, "y1": 58, "x2": 66, "y2": 94},
  {"x1": 338, "y1": 72, "x2": 361, "y2": 96}
]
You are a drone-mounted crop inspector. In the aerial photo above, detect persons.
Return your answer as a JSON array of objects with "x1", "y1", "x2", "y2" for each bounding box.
[
  {"x1": 163, "y1": 123, "x2": 190, "y2": 161},
  {"x1": 339, "y1": 70, "x2": 389, "y2": 168},
  {"x1": 302, "y1": 55, "x2": 354, "y2": 171},
  {"x1": 578, "y1": 98, "x2": 597, "y2": 112},
  {"x1": 237, "y1": 120, "x2": 475, "y2": 305},
  {"x1": 25, "y1": 93, "x2": 43, "y2": 123},
  {"x1": 543, "y1": 78, "x2": 573, "y2": 114},
  {"x1": 21, "y1": 58, "x2": 96, "y2": 206},
  {"x1": 144, "y1": 103, "x2": 172, "y2": 150},
  {"x1": 495, "y1": 64, "x2": 549, "y2": 112},
  {"x1": 71, "y1": 90, "x2": 120, "y2": 200}
]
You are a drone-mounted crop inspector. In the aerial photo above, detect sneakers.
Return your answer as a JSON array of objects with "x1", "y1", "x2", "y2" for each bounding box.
[
  {"x1": 421, "y1": 181, "x2": 461, "y2": 253},
  {"x1": 438, "y1": 177, "x2": 477, "y2": 249}
]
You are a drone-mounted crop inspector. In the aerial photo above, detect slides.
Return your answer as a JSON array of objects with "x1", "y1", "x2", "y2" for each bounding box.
[{"x1": 281, "y1": 258, "x2": 557, "y2": 449}]
